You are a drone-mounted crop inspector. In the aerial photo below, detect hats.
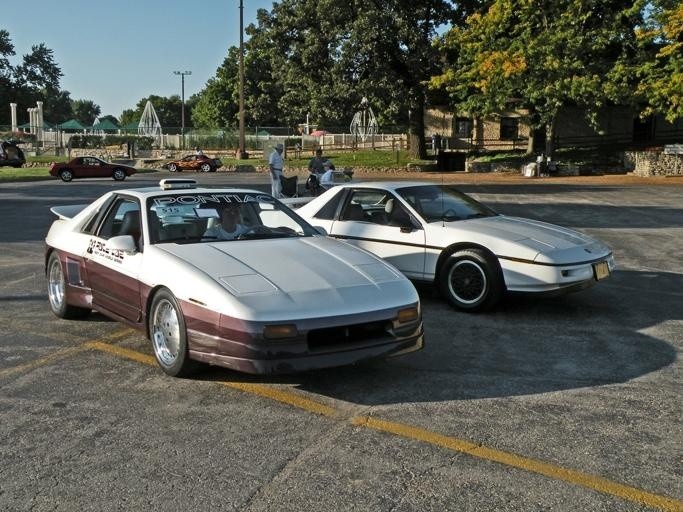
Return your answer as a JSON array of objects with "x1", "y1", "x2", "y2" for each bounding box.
[
  {"x1": 322, "y1": 161, "x2": 331, "y2": 165},
  {"x1": 274, "y1": 144, "x2": 284, "y2": 149}
]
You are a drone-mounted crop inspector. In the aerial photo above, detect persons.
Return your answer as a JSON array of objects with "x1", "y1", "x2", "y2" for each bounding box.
[
  {"x1": 240, "y1": 149, "x2": 247, "y2": 160},
  {"x1": 315, "y1": 160, "x2": 335, "y2": 198},
  {"x1": 268, "y1": 144, "x2": 284, "y2": 198},
  {"x1": 307, "y1": 148, "x2": 327, "y2": 196},
  {"x1": 534, "y1": 153, "x2": 549, "y2": 178},
  {"x1": 194, "y1": 146, "x2": 202, "y2": 172},
  {"x1": 199, "y1": 199, "x2": 255, "y2": 241},
  {"x1": 235, "y1": 149, "x2": 241, "y2": 159}
]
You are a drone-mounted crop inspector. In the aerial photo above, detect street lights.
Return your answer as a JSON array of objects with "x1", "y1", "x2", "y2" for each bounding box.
[
  {"x1": 172, "y1": 70, "x2": 191, "y2": 151},
  {"x1": 359, "y1": 96, "x2": 369, "y2": 149}
]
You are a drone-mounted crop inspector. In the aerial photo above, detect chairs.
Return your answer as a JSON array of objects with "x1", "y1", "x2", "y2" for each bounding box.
[
  {"x1": 345, "y1": 199, "x2": 423, "y2": 227},
  {"x1": 111, "y1": 200, "x2": 222, "y2": 245}
]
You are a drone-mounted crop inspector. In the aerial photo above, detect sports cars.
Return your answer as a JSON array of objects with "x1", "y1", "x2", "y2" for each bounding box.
[
  {"x1": 245, "y1": 180, "x2": 618, "y2": 314},
  {"x1": 40, "y1": 174, "x2": 428, "y2": 380},
  {"x1": 161, "y1": 153, "x2": 223, "y2": 174},
  {"x1": 47, "y1": 155, "x2": 138, "y2": 183}
]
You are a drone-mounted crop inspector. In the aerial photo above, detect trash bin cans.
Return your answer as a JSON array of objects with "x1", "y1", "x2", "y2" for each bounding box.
[{"x1": 432, "y1": 135, "x2": 440, "y2": 151}]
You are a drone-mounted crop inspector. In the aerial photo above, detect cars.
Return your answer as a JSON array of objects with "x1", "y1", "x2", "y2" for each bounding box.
[
  {"x1": 65, "y1": 134, "x2": 100, "y2": 149},
  {"x1": 0, "y1": 136, "x2": 27, "y2": 168}
]
